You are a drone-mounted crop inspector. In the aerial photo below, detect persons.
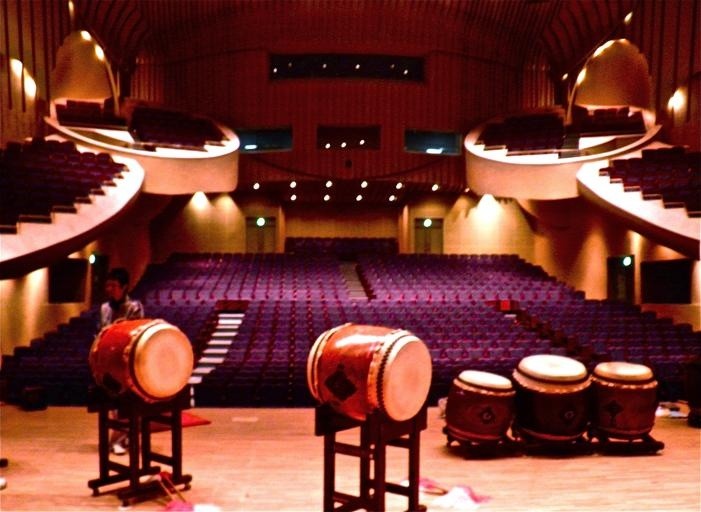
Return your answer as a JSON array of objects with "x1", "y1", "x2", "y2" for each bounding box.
[{"x1": 98, "y1": 268, "x2": 144, "y2": 439}]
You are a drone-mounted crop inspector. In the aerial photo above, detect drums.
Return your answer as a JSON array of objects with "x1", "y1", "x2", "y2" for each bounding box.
[
  {"x1": 512, "y1": 354, "x2": 591, "y2": 440},
  {"x1": 90, "y1": 318, "x2": 194, "y2": 404},
  {"x1": 306, "y1": 323, "x2": 432, "y2": 422},
  {"x1": 445, "y1": 370, "x2": 516, "y2": 440},
  {"x1": 592, "y1": 361, "x2": 658, "y2": 434}
]
